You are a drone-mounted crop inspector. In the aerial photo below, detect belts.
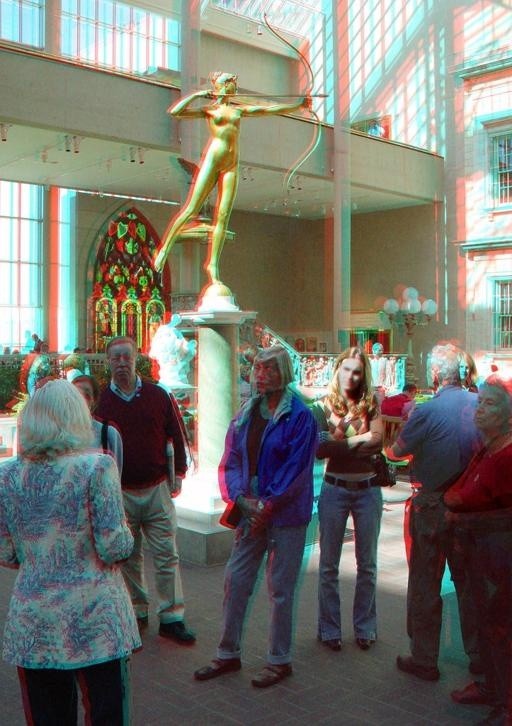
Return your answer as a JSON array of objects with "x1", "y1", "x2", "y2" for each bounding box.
[{"x1": 325, "y1": 476, "x2": 377, "y2": 490}]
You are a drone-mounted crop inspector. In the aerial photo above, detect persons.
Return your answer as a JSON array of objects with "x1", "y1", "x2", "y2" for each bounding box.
[
  {"x1": 150, "y1": 69, "x2": 311, "y2": 285},
  {"x1": 368, "y1": 120, "x2": 385, "y2": 137},
  {"x1": 0, "y1": 333, "x2": 196, "y2": 726},
  {"x1": 194, "y1": 346, "x2": 316, "y2": 690},
  {"x1": 312, "y1": 341, "x2": 512, "y2": 726}
]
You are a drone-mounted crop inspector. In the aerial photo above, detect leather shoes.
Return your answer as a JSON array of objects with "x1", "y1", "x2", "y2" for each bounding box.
[
  {"x1": 358, "y1": 637, "x2": 372, "y2": 649},
  {"x1": 397, "y1": 655, "x2": 439, "y2": 680},
  {"x1": 160, "y1": 622, "x2": 195, "y2": 642},
  {"x1": 250, "y1": 664, "x2": 291, "y2": 687},
  {"x1": 327, "y1": 638, "x2": 342, "y2": 650},
  {"x1": 194, "y1": 657, "x2": 242, "y2": 679},
  {"x1": 451, "y1": 683, "x2": 500, "y2": 705}
]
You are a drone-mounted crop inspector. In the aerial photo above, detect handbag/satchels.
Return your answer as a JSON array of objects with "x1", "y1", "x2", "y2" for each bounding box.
[{"x1": 376, "y1": 455, "x2": 396, "y2": 485}]
